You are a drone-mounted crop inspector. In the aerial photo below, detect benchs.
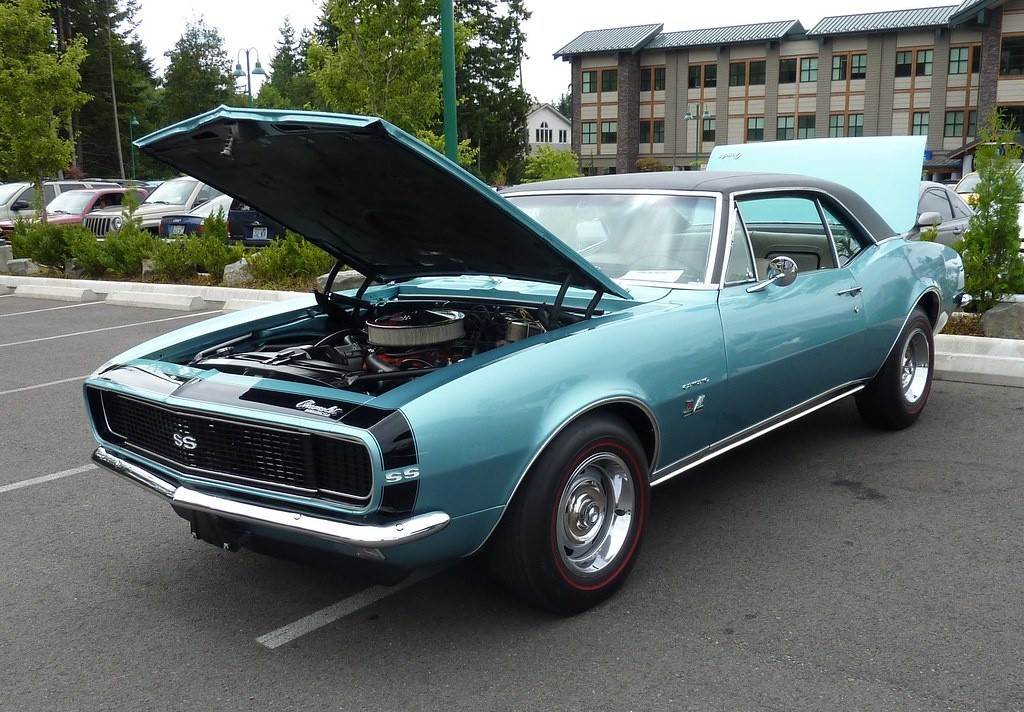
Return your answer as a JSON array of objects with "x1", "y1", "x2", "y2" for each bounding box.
[{"x1": 619, "y1": 231, "x2": 846, "y2": 278}]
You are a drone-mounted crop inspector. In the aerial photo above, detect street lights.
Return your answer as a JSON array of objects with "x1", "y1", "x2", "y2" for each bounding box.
[
  {"x1": 227, "y1": 47, "x2": 267, "y2": 108},
  {"x1": 684, "y1": 98, "x2": 711, "y2": 171},
  {"x1": 129, "y1": 108, "x2": 140, "y2": 180}
]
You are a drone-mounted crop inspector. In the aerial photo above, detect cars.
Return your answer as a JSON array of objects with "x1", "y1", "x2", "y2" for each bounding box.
[
  {"x1": 81, "y1": 101, "x2": 974, "y2": 617},
  {"x1": 951, "y1": 170, "x2": 1013, "y2": 210},
  {"x1": 974, "y1": 163, "x2": 1024, "y2": 253},
  {"x1": 900, "y1": 181, "x2": 984, "y2": 254}
]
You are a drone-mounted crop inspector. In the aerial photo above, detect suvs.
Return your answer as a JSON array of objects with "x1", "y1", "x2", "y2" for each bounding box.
[{"x1": 0, "y1": 174, "x2": 286, "y2": 248}]
